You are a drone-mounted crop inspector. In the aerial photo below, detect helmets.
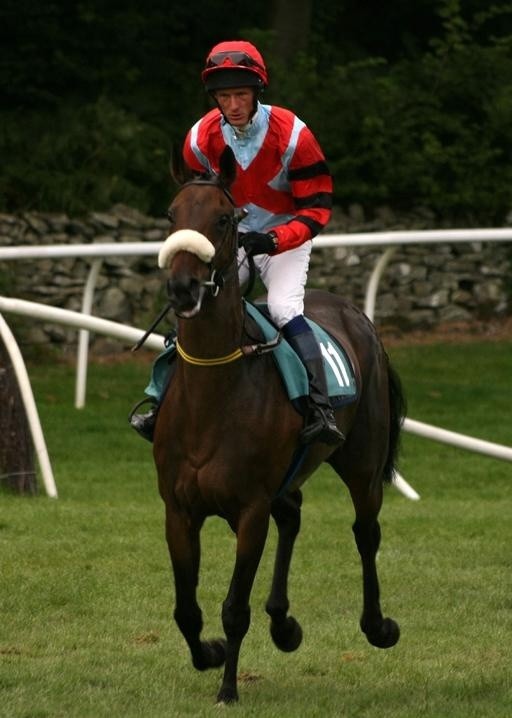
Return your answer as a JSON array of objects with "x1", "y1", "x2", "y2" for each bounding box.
[{"x1": 201, "y1": 41, "x2": 269, "y2": 98}]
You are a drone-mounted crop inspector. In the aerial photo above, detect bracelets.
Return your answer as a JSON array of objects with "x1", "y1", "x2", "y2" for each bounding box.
[{"x1": 269, "y1": 232, "x2": 278, "y2": 250}]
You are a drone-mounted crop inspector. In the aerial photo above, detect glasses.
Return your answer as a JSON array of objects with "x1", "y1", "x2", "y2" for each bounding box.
[{"x1": 208, "y1": 50, "x2": 263, "y2": 71}]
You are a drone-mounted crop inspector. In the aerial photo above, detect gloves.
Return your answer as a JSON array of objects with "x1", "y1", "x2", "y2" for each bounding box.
[{"x1": 238, "y1": 231, "x2": 275, "y2": 257}]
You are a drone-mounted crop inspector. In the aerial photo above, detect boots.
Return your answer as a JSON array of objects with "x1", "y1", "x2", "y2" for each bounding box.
[
  {"x1": 131, "y1": 406, "x2": 158, "y2": 443},
  {"x1": 281, "y1": 315, "x2": 345, "y2": 446}
]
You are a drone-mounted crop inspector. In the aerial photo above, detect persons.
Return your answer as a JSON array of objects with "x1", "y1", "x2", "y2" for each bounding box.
[{"x1": 130, "y1": 40, "x2": 347, "y2": 446}]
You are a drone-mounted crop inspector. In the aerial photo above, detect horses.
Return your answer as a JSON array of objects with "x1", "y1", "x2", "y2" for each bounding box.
[{"x1": 152, "y1": 143, "x2": 405, "y2": 709}]
